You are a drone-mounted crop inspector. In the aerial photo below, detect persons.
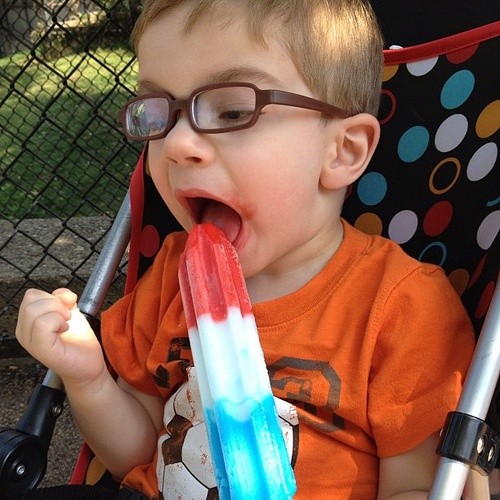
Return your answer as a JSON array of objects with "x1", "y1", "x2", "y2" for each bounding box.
[{"x1": 16, "y1": 1, "x2": 496, "y2": 500}]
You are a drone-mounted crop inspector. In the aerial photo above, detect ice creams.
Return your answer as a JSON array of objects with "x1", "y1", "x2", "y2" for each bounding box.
[{"x1": 178, "y1": 222, "x2": 297, "y2": 500}]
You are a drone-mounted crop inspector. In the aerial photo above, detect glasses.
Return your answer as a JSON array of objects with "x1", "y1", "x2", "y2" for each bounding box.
[{"x1": 118, "y1": 81, "x2": 350, "y2": 141}]
